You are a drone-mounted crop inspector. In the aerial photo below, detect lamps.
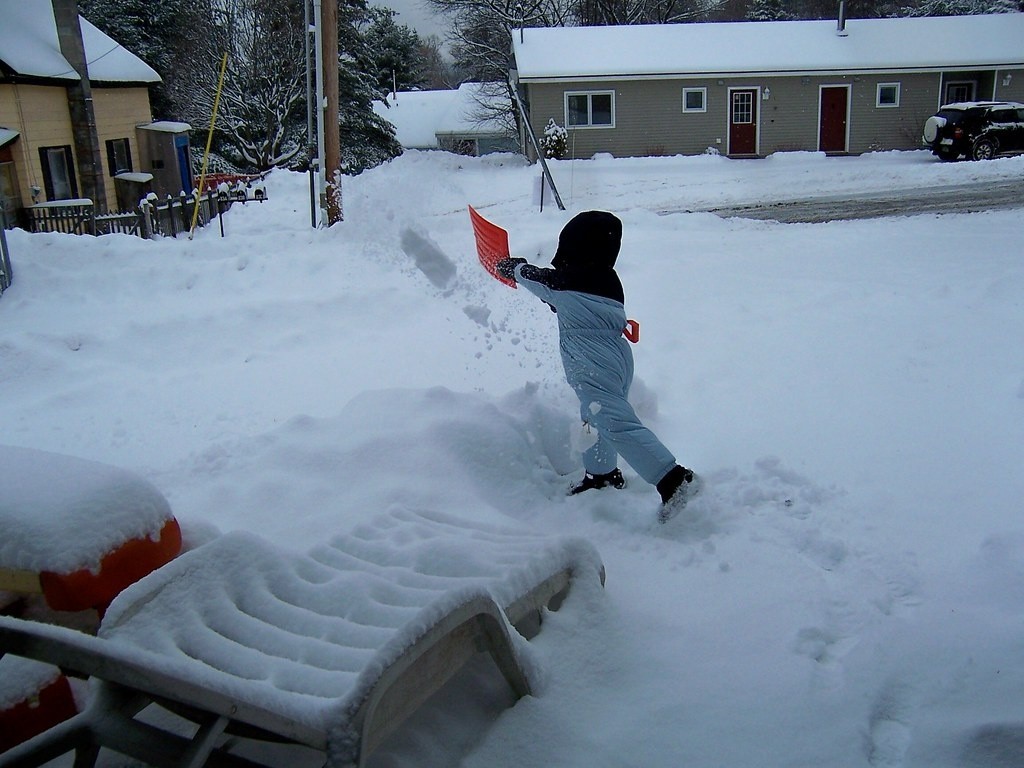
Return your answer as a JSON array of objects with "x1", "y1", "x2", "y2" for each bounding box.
[
  {"x1": 1003, "y1": 74, "x2": 1012, "y2": 86},
  {"x1": 762, "y1": 86, "x2": 770, "y2": 100}
]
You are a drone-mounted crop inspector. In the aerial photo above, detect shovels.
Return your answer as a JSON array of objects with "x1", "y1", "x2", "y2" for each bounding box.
[{"x1": 466, "y1": 204, "x2": 640, "y2": 343}]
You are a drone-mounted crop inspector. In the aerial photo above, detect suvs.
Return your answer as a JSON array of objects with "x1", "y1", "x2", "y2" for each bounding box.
[{"x1": 922, "y1": 102, "x2": 1024, "y2": 162}]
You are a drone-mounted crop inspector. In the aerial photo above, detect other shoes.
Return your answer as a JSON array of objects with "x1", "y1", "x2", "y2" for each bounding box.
[
  {"x1": 655, "y1": 465, "x2": 705, "y2": 524},
  {"x1": 565, "y1": 468, "x2": 626, "y2": 496}
]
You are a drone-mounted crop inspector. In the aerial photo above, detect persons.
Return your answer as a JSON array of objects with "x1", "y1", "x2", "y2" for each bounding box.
[{"x1": 495, "y1": 211, "x2": 704, "y2": 523}]
